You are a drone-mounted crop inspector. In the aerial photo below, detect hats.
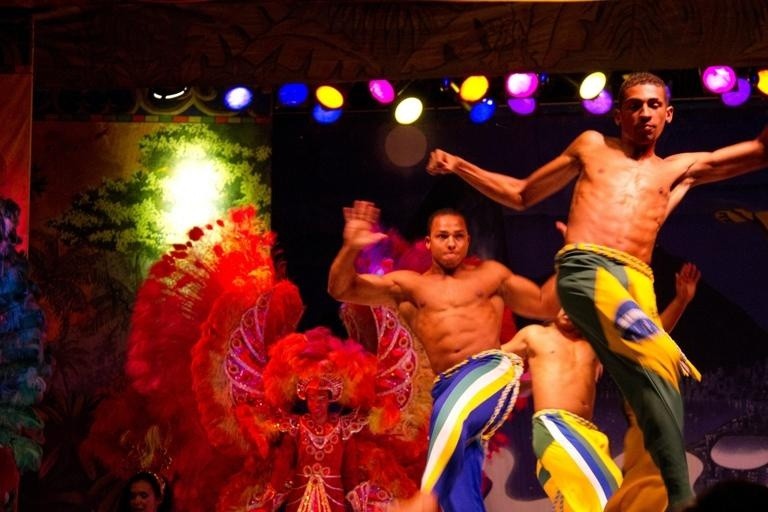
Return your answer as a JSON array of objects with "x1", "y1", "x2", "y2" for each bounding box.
[{"x1": 296, "y1": 374, "x2": 345, "y2": 402}]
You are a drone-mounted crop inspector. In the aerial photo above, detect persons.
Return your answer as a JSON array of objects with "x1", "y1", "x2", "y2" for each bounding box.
[
  {"x1": 257, "y1": 385, "x2": 376, "y2": 512},
  {"x1": 498, "y1": 259, "x2": 704, "y2": 512},
  {"x1": 114, "y1": 468, "x2": 173, "y2": 512},
  {"x1": 326, "y1": 198, "x2": 574, "y2": 511},
  {"x1": 425, "y1": 70, "x2": 767, "y2": 512}
]
